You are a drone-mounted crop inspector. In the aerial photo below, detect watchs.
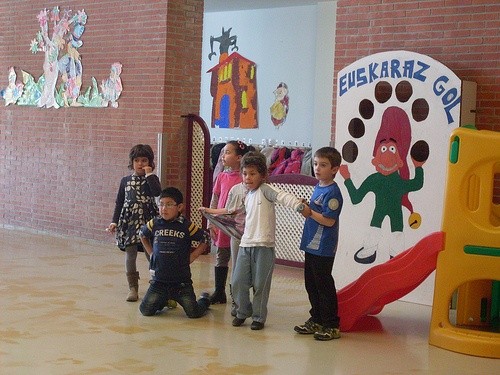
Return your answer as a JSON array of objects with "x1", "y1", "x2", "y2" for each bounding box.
[{"x1": 296, "y1": 202, "x2": 306, "y2": 213}]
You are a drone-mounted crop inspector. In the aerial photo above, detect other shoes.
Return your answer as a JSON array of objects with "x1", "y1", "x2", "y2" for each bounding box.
[
  {"x1": 167, "y1": 299, "x2": 177, "y2": 310},
  {"x1": 231, "y1": 302, "x2": 239, "y2": 317},
  {"x1": 200, "y1": 291, "x2": 214, "y2": 307},
  {"x1": 232, "y1": 318, "x2": 245, "y2": 326},
  {"x1": 251, "y1": 321, "x2": 264, "y2": 330}
]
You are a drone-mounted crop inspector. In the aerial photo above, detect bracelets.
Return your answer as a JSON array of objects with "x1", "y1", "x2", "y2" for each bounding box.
[{"x1": 305, "y1": 207, "x2": 313, "y2": 218}]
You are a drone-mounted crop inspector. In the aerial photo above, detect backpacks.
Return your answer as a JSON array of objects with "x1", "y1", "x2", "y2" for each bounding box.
[{"x1": 259, "y1": 146, "x2": 316, "y2": 178}]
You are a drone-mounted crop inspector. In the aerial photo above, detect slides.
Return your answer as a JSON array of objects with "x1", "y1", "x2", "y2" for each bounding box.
[{"x1": 337, "y1": 230, "x2": 445, "y2": 332}]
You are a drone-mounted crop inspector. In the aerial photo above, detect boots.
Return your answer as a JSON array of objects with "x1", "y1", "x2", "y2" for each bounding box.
[
  {"x1": 209, "y1": 266, "x2": 229, "y2": 304},
  {"x1": 126, "y1": 272, "x2": 141, "y2": 301}
]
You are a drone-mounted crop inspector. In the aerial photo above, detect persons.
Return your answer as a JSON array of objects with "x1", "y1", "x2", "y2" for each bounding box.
[
  {"x1": 197, "y1": 138, "x2": 345, "y2": 341},
  {"x1": 138, "y1": 185, "x2": 213, "y2": 320},
  {"x1": 105, "y1": 143, "x2": 163, "y2": 303}
]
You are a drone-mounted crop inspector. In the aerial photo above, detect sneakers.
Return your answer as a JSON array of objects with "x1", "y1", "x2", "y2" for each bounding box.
[
  {"x1": 313, "y1": 327, "x2": 342, "y2": 340},
  {"x1": 294, "y1": 318, "x2": 324, "y2": 334}
]
